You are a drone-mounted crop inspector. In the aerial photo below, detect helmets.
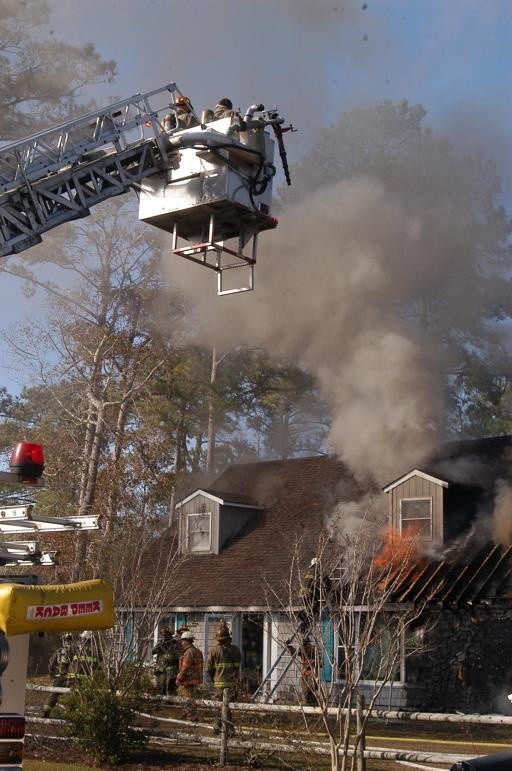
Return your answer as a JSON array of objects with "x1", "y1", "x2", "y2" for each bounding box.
[
  {"x1": 215, "y1": 626, "x2": 230, "y2": 641},
  {"x1": 181, "y1": 631, "x2": 195, "y2": 640},
  {"x1": 161, "y1": 625, "x2": 189, "y2": 637}
]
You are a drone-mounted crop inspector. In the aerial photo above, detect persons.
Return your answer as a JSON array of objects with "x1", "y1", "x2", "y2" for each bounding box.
[
  {"x1": 41, "y1": 629, "x2": 103, "y2": 718},
  {"x1": 151, "y1": 625, "x2": 203, "y2": 721},
  {"x1": 160, "y1": 96, "x2": 242, "y2": 132},
  {"x1": 298, "y1": 558, "x2": 333, "y2": 626},
  {"x1": 206, "y1": 628, "x2": 242, "y2": 734}
]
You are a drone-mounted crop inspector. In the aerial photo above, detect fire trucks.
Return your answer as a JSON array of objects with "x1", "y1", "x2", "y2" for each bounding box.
[{"x1": 0, "y1": 82, "x2": 290, "y2": 771}]
